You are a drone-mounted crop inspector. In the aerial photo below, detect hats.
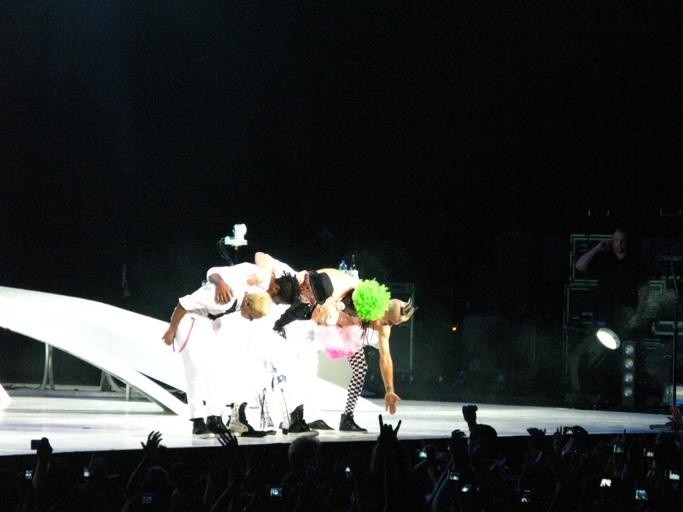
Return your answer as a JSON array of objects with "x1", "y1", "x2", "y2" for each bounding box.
[{"x1": 309, "y1": 271, "x2": 334, "y2": 305}]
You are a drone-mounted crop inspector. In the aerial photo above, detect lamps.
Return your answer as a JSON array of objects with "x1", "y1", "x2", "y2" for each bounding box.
[
  {"x1": 621, "y1": 340, "x2": 638, "y2": 409},
  {"x1": 594, "y1": 304, "x2": 634, "y2": 351}
]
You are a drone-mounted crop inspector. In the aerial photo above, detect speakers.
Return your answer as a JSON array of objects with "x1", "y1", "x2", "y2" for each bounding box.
[
  {"x1": 569, "y1": 234, "x2": 618, "y2": 284},
  {"x1": 364, "y1": 281, "x2": 414, "y2": 398},
  {"x1": 561, "y1": 285, "x2": 614, "y2": 404},
  {"x1": 637, "y1": 339, "x2": 683, "y2": 415}
]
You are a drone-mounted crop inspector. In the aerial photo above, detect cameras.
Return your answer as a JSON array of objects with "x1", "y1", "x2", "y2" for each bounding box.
[
  {"x1": 613, "y1": 444, "x2": 624, "y2": 453},
  {"x1": 25, "y1": 469, "x2": 33, "y2": 480},
  {"x1": 268, "y1": 486, "x2": 283, "y2": 497},
  {"x1": 83, "y1": 471, "x2": 91, "y2": 477},
  {"x1": 668, "y1": 469, "x2": 680, "y2": 481},
  {"x1": 633, "y1": 489, "x2": 649, "y2": 501},
  {"x1": 31, "y1": 440, "x2": 41, "y2": 449},
  {"x1": 448, "y1": 470, "x2": 461, "y2": 482},
  {"x1": 645, "y1": 451, "x2": 655, "y2": 457},
  {"x1": 599, "y1": 477, "x2": 613, "y2": 487},
  {"x1": 140, "y1": 493, "x2": 155, "y2": 504},
  {"x1": 417, "y1": 450, "x2": 429, "y2": 458}
]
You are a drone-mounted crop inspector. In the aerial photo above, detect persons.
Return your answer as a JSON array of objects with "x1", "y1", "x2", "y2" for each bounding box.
[
  {"x1": 576, "y1": 227, "x2": 649, "y2": 329},
  {"x1": 161, "y1": 251, "x2": 417, "y2": 439},
  {"x1": 1, "y1": 405, "x2": 683, "y2": 511}
]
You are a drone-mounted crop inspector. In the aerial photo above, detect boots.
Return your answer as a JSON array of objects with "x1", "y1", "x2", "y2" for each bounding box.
[
  {"x1": 193, "y1": 403, "x2": 335, "y2": 437},
  {"x1": 339, "y1": 414, "x2": 367, "y2": 434}
]
